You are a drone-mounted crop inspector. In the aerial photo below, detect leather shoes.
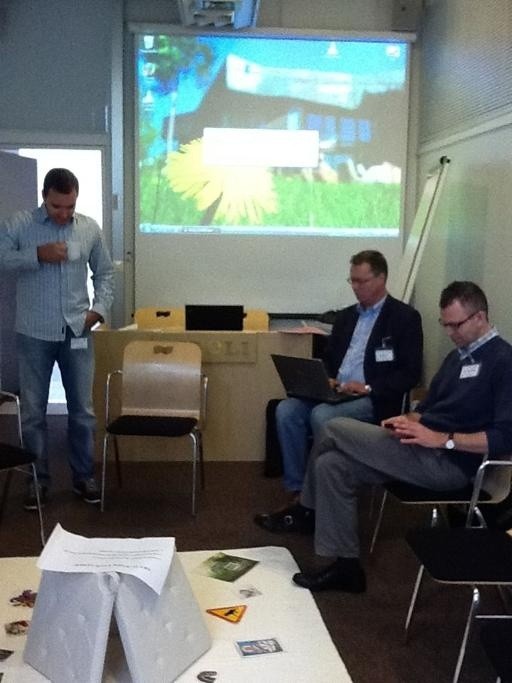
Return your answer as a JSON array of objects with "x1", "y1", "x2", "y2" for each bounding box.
[
  {"x1": 254, "y1": 504, "x2": 314, "y2": 535},
  {"x1": 293, "y1": 561, "x2": 368, "y2": 594}
]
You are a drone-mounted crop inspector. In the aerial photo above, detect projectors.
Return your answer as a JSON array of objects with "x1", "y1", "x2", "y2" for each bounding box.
[{"x1": 177, "y1": 0, "x2": 258, "y2": 30}]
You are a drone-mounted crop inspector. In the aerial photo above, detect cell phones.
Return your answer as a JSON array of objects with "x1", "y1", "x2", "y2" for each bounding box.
[{"x1": 385, "y1": 423, "x2": 396, "y2": 429}]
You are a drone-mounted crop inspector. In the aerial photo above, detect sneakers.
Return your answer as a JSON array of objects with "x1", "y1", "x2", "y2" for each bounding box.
[
  {"x1": 24, "y1": 484, "x2": 46, "y2": 510},
  {"x1": 71, "y1": 476, "x2": 101, "y2": 504}
]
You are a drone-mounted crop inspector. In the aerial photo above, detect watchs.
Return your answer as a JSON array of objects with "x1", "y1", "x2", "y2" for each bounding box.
[
  {"x1": 364, "y1": 382, "x2": 371, "y2": 395},
  {"x1": 441, "y1": 430, "x2": 456, "y2": 451}
]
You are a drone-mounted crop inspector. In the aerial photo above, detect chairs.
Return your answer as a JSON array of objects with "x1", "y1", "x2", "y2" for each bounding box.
[
  {"x1": 393, "y1": 456, "x2": 511, "y2": 682},
  {"x1": 362, "y1": 426, "x2": 493, "y2": 576},
  {"x1": 97, "y1": 338, "x2": 212, "y2": 521},
  {"x1": 0, "y1": 389, "x2": 50, "y2": 551}
]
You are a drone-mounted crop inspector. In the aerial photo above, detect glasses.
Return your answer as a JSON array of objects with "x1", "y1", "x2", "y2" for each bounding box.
[
  {"x1": 438, "y1": 314, "x2": 476, "y2": 329},
  {"x1": 347, "y1": 276, "x2": 371, "y2": 285}
]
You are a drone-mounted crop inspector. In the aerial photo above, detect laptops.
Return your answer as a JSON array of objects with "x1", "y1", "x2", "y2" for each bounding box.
[{"x1": 270, "y1": 353, "x2": 364, "y2": 405}]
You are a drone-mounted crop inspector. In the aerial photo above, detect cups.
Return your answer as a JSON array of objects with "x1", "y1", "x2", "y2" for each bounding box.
[{"x1": 65, "y1": 239, "x2": 83, "y2": 260}]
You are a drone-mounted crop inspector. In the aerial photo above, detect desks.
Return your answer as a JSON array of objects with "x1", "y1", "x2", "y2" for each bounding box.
[
  {"x1": 0, "y1": 542, "x2": 355, "y2": 682},
  {"x1": 84, "y1": 318, "x2": 317, "y2": 465}
]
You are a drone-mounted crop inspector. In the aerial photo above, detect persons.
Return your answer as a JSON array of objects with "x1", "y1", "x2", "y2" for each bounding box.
[
  {"x1": 253, "y1": 280, "x2": 511, "y2": 594},
  {"x1": 274, "y1": 249, "x2": 424, "y2": 494},
  {"x1": 1, "y1": 167, "x2": 117, "y2": 510}
]
